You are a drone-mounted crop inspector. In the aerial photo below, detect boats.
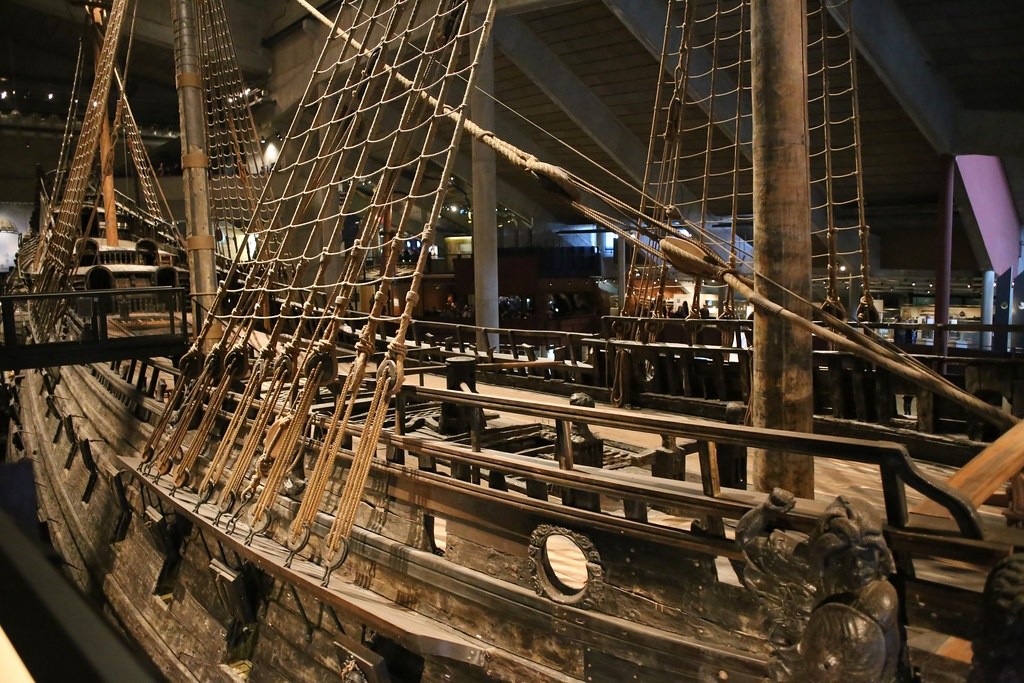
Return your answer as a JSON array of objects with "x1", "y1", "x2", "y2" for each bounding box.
[{"x1": 0, "y1": 0, "x2": 1024, "y2": 683}]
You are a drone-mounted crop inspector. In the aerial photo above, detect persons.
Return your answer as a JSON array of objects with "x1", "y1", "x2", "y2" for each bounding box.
[
  {"x1": 650, "y1": 298, "x2": 711, "y2": 320},
  {"x1": 893, "y1": 314, "x2": 907, "y2": 344}
]
